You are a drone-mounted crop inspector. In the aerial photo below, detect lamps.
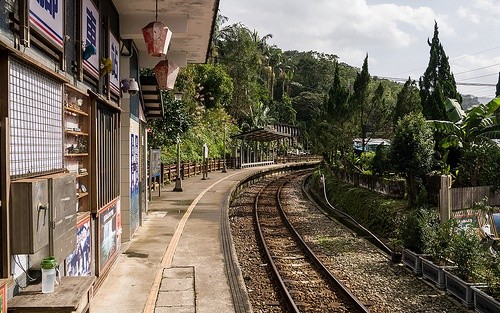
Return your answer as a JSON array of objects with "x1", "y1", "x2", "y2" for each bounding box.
[
  {"x1": 141, "y1": 0, "x2": 172, "y2": 58},
  {"x1": 153, "y1": 55, "x2": 180, "y2": 91}
]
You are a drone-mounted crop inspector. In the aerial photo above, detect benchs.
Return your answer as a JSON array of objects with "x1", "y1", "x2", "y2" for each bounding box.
[{"x1": 242, "y1": 160, "x2": 273, "y2": 169}]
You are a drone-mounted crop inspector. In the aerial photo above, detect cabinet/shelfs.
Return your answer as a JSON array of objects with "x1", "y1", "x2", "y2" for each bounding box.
[{"x1": 62, "y1": 83, "x2": 92, "y2": 222}]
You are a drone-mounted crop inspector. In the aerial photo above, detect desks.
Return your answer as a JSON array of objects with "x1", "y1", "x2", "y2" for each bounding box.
[{"x1": 7, "y1": 274, "x2": 97, "y2": 313}]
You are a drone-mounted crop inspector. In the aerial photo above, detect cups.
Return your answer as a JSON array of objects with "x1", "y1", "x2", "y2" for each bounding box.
[{"x1": 41, "y1": 256, "x2": 56, "y2": 293}]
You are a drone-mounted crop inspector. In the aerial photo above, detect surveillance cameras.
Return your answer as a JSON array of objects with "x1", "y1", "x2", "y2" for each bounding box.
[{"x1": 128, "y1": 78, "x2": 139, "y2": 96}]
